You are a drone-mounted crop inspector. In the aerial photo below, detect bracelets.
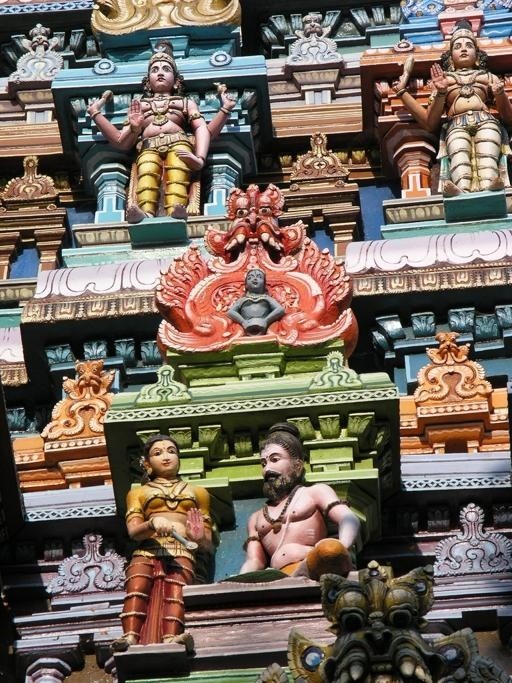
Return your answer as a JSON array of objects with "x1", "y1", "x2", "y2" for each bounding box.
[
  {"x1": 221, "y1": 106, "x2": 231, "y2": 115},
  {"x1": 131, "y1": 125, "x2": 139, "y2": 132},
  {"x1": 497, "y1": 89, "x2": 505, "y2": 95},
  {"x1": 148, "y1": 516, "x2": 155, "y2": 529},
  {"x1": 90, "y1": 111, "x2": 101, "y2": 120},
  {"x1": 397, "y1": 88, "x2": 407, "y2": 96},
  {"x1": 197, "y1": 155, "x2": 205, "y2": 162},
  {"x1": 437, "y1": 90, "x2": 448, "y2": 98}
]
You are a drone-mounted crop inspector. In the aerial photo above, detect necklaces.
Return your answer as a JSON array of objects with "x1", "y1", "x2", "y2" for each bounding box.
[
  {"x1": 154, "y1": 477, "x2": 179, "y2": 487},
  {"x1": 262, "y1": 484, "x2": 303, "y2": 533}
]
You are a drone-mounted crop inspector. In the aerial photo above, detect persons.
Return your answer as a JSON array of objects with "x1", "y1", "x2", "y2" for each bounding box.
[
  {"x1": 109, "y1": 434, "x2": 217, "y2": 654},
  {"x1": 395, "y1": 17, "x2": 511, "y2": 196},
  {"x1": 237, "y1": 422, "x2": 361, "y2": 580},
  {"x1": 226, "y1": 267, "x2": 285, "y2": 331},
  {"x1": 86, "y1": 38, "x2": 238, "y2": 223}
]
